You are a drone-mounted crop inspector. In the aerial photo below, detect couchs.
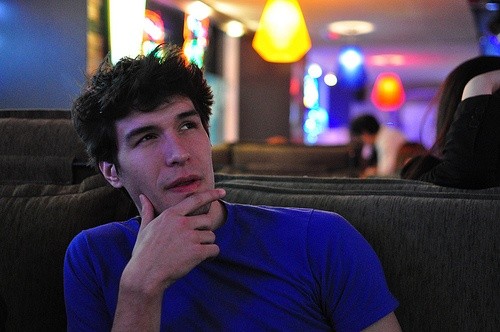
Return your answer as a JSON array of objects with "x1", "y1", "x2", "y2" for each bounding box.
[{"x1": 0, "y1": 106, "x2": 500, "y2": 332}]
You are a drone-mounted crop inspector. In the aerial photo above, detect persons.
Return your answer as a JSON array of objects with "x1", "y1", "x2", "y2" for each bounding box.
[
  {"x1": 64, "y1": 41, "x2": 402, "y2": 332},
  {"x1": 350, "y1": 114, "x2": 404, "y2": 179},
  {"x1": 401, "y1": 13, "x2": 500, "y2": 191}
]
